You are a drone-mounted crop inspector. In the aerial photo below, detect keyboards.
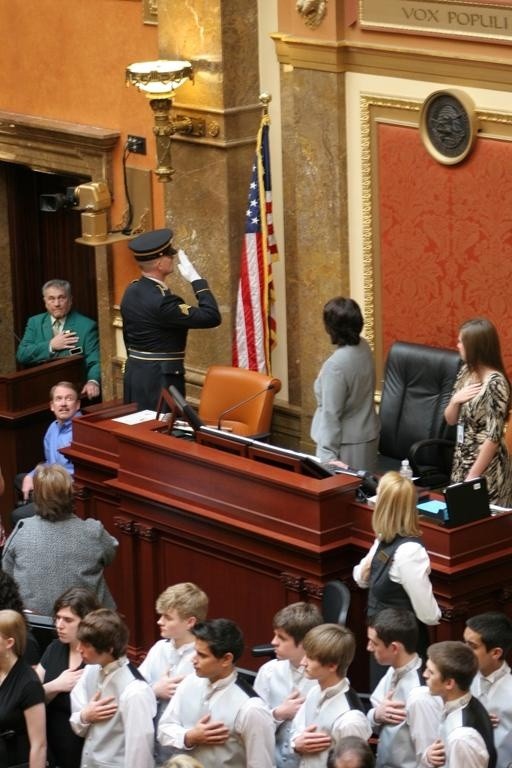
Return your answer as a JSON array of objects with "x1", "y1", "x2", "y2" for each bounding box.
[{"x1": 321, "y1": 463, "x2": 375, "y2": 483}]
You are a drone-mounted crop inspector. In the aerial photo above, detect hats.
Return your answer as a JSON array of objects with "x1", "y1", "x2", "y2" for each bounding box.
[{"x1": 128, "y1": 229, "x2": 176, "y2": 262}]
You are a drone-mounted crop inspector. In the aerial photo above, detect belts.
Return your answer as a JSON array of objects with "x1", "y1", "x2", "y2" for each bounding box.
[{"x1": 128, "y1": 348, "x2": 184, "y2": 360}]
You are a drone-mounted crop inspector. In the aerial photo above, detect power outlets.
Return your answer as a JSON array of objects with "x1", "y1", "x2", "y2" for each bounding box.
[{"x1": 127, "y1": 135, "x2": 146, "y2": 155}]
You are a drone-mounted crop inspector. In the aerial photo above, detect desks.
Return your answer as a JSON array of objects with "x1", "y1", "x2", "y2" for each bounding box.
[{"x1": 56, "y1": 401, "x2": 512, "y2": 709}]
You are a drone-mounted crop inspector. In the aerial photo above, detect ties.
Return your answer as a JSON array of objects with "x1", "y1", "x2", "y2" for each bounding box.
[{"x1": 53, "y1": 321, "x2": 61, "y2": 336}]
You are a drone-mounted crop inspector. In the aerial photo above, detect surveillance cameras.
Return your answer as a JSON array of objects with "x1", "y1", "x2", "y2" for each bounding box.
[{"x1": 40, "y1": 186, "x2": 78, "y2": 212}]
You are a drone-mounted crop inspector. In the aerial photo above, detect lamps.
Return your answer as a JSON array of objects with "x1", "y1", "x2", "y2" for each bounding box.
[{"x1": 124, "y1": 60, "x2": 196, "y2": 183}]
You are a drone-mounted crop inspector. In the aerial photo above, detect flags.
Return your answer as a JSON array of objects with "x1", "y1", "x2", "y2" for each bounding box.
[{"x1": 232, "y1": 114, "x2": 280, "y2": 378}]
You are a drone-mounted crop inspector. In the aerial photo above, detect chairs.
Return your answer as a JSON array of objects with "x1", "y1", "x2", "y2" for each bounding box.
[
  {"x1": 377, "y1": 340, "x2": 464, "y2": 490},
  {"x1": 251, "y1": 578, "x2": 354, "y2": 660},
  {"x1": 197, "y1": 365, "x2": 282, "y2": 444},
  {"x1": 16, "y1": 409, "x2": 88, "y2": 509}
]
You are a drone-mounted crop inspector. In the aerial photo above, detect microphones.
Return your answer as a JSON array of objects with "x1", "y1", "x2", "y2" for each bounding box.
[
  {"x1": 218, "y1": 384, "x2": 274, "y2": 430},
  {"x1": 0, "y1": 521, "x2": 24, "y2": 560}
]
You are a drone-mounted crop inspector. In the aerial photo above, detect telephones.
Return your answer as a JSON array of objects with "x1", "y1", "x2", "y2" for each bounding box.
[{"x1": 333, "y1": 467, "x2": 379, "y2": 496}]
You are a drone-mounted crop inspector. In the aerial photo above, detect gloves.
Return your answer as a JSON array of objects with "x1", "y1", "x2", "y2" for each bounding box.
[{"x1": 176, "y1": 249, "x2": 201, "y2": 283}]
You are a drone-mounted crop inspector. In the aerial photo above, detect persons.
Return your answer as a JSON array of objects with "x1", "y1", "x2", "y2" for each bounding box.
[
  {"x1": 137, "y1": 582, "x2": 277, "y2": 768},
  {"x1": 11, "y1": 381, "x2": 84, "y2": 526},
  {"x1": 353, "y1": 471, "x2": 442, "y2": 694},
  {"x1": 0, "y1": 571, "x2": 47, "y2": 768},
  {"x1": 121, "y1": 229, "x2": 221, "y2": 417},
  {"x1": 445, "y1": 320, "x2": 512, "y2": 510},
  {"x1": 17, "y1": 280, "x2": 101, "y2": 400},
  {"x1": 35, "y1": 586, "x2": 158, "y2": 767},
  {"x1": 364, "y1": 606, "x2": 512, "y2": 767},
  {"x1": 311, "y1": 297, "x2": 382, "y2": 473},
  {"x1": 254, "y1": 601, "x2": 375, "y2": 766},
  {"x1": 1, "y1": 466, "x2": 119, "y2": 642}
]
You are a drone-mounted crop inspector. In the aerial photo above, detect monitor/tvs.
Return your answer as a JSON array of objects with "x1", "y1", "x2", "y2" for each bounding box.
[
  {"x1": 199, "y1": 425, "x2": 253, "y2": 448},
  {"x1": 252, "y1": 440, "x2": 335, "y2": 480},
  {"x1": 169, "y1": 383, "x2": 203, "y2": 433}
]
